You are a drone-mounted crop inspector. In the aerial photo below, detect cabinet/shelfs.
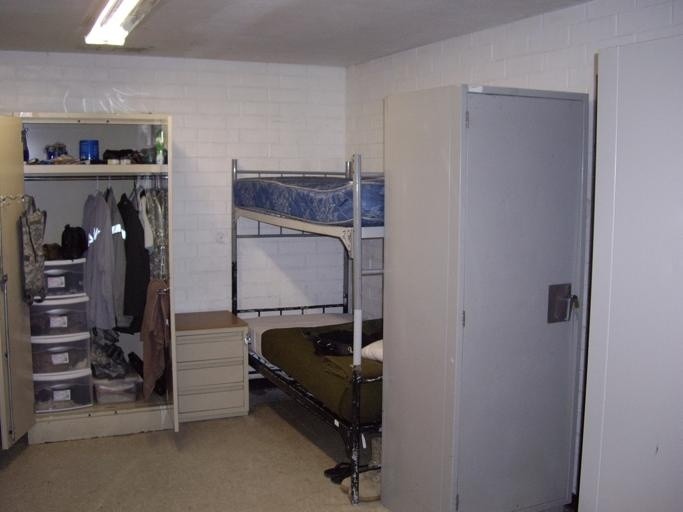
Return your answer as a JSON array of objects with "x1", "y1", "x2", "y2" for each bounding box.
[
  {"x1": 382, "y1": 83, "x2": 588, "y2": 511},
  {"x1": 1, "y1": 113, "x2": 177, "y2": 454}
]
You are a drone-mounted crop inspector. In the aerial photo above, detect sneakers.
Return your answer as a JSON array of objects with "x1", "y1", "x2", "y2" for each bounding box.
[{"x1": 341, "y1": 469, "x2": 380, "y2": 502}]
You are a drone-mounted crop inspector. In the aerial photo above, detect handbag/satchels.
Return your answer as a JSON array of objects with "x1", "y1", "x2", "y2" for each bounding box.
[
  {"x1": 313, "y1": 330, "x2": 367, "y2": 355},
  {"x1": 20, "y1": 197, "x2": 46, "y2": 306}
]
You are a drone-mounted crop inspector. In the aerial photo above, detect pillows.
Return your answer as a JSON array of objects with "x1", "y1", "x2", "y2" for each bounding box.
[{"x1": 357, "y1": 339, "x2": 383, "y2": 363}]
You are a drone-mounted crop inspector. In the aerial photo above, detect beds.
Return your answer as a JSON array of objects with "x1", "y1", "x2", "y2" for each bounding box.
[{"x1": 231, "y1": 156, "x2": 384, "y2": 506}]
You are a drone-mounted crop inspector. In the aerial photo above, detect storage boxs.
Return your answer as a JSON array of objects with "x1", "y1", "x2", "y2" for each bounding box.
[{"x1": 27, "y1": 259, "x2": 144, "y2": 416}]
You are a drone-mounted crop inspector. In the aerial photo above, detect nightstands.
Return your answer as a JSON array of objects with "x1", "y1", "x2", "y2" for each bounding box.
[{"x1": 175, "y1": 311, "x2": 249, "y2": 426}]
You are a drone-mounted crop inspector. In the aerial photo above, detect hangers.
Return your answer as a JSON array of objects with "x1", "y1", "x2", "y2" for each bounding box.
[{"x1": 91, "y1": 174, "x2": 165, "y2": 194}]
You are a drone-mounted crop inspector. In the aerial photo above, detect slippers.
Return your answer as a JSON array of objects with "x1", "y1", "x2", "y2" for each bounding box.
[{"x1": 325, "y1": 463, "x2": 381, "y2": 487}]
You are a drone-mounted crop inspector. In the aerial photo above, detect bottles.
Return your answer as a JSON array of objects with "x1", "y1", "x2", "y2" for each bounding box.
[{"x1": 155, "y1": 128, "x2": 165, "y2": 165}]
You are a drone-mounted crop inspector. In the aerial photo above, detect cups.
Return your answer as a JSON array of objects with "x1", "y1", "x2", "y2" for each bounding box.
[{"x1": 78, "y1": 139, "x2": 98, "y2": 163}]
